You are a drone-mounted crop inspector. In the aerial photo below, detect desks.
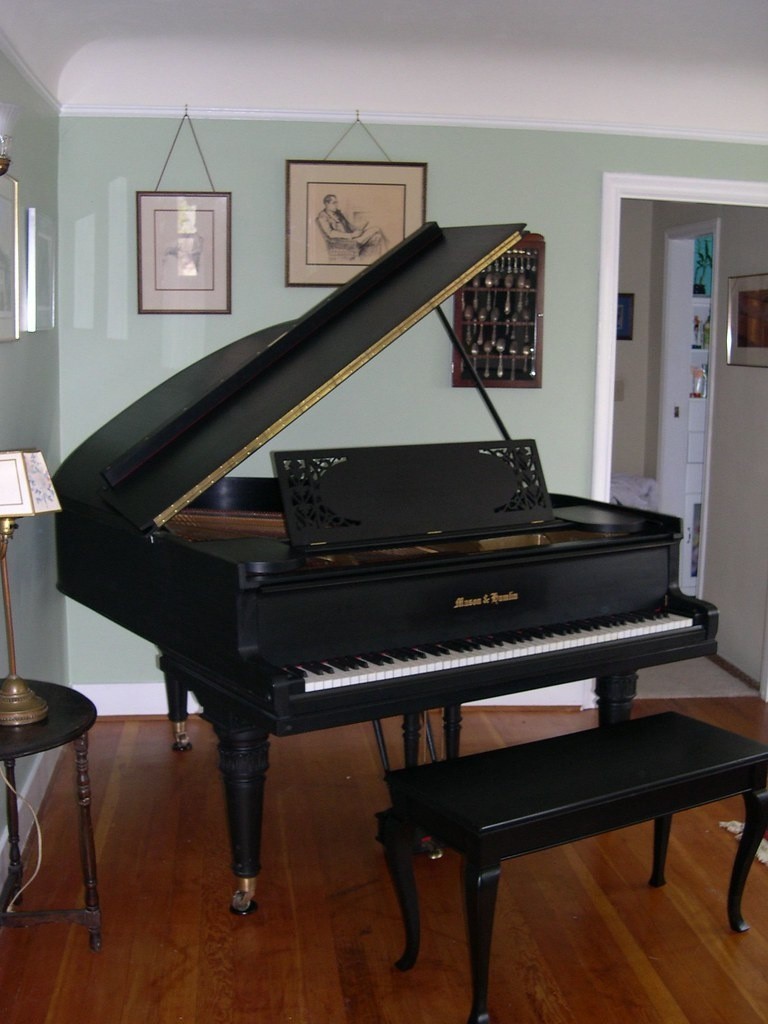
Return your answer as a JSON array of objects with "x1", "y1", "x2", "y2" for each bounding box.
[{"x1": 0, "y1": 678, "x2": 104, "y2": 953}]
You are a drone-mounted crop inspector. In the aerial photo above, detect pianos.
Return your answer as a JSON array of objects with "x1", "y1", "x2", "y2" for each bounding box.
[{"x1": 49, "y1": 220, "x2": 722, "y2": 917}]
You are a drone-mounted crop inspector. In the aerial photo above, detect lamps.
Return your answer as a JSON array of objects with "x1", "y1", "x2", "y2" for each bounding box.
[{"x1": 1, "y1": 448, "x2": 63, "y2": 915}]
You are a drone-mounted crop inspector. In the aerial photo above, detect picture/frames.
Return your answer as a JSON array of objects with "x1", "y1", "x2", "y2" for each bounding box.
[
  {"x1": 135, "y1": 114, "x2": 232, "y2": 314},
  {"x1": 0, "y1": 173, "x2": 20, "y2": 344},
  {"x1": 725, "y1": 273, "x2": 768, "y2": 369},
  {"x1": 284, "y1": 118, "x2": 428, "y2": 288},
  {"x1": 26, "y1": 207, "x2": 58, "y2": 333},
  {"x1": 615, "y1": 293, "x2": 635, "y2": 341}
]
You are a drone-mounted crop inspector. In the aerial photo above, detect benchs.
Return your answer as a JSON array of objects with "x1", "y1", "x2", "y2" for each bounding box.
[{"x1": 371, "y1": 709, "x2": 768, "y2": 1023}]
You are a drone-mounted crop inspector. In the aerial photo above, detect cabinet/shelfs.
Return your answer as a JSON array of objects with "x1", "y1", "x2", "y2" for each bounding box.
[
  {"x1": 676, "y1": 294, "x2": 713, "y2": 598},
  {"x1": 451, "y1": 230, "x2": 546, "y2": 390}
]
